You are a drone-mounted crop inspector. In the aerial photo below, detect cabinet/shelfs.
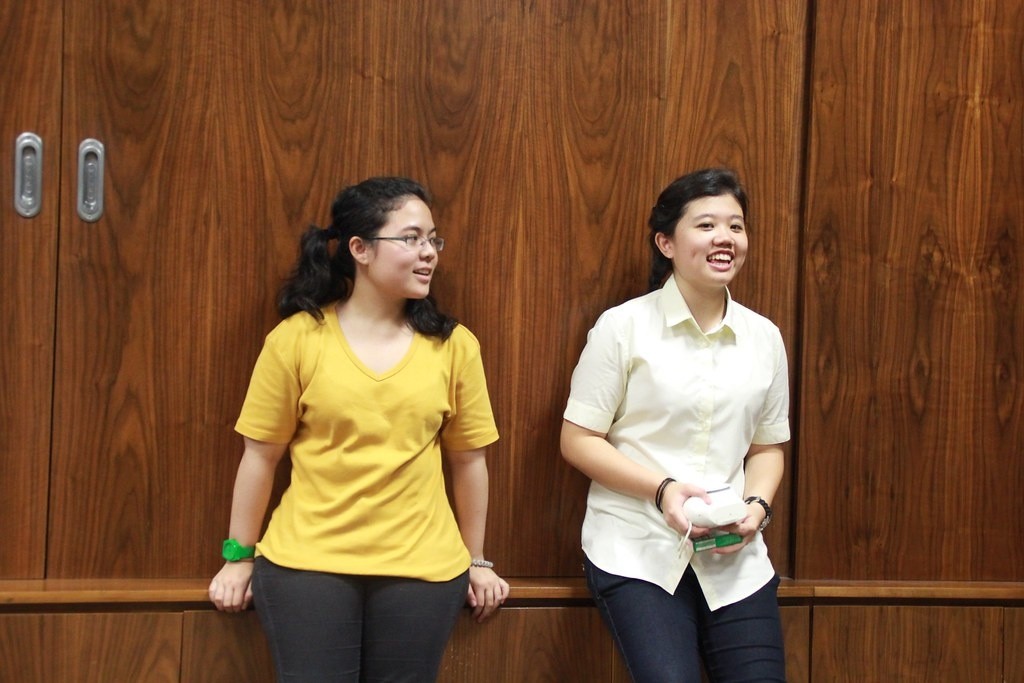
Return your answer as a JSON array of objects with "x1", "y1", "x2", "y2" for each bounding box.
[{"x1": 0, "y1": 1, "x2": 1024, "y2": 682}]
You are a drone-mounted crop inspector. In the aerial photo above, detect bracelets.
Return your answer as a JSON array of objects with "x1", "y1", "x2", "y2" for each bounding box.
[
  {"x1": 471, "y1": 560, "x2": 493, "y2": 567},
  {"x1": 655, "y1": 478, "x2": 675, "y2": 513}
]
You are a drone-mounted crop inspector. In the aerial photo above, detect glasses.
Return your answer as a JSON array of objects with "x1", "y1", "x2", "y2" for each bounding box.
[{"x1": 368, "y1": 235, "x2": 443, "y2": 252}]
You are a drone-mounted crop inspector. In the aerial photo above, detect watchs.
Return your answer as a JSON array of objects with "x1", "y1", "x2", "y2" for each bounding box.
[
  {"x1": 744, "y1": 496, "x2": 772, "y2": 531},
  {"x1": 222, "y1": 539, "x2": 254, "y2": 562}
]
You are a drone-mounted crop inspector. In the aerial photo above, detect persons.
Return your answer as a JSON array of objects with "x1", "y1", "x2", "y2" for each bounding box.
[
  {"x1": 560, "y1": 168, "x2": 791, "y2": 683},
  {"x1": 208, "y1": 178, "x2": 509, "y2": 683}
]
